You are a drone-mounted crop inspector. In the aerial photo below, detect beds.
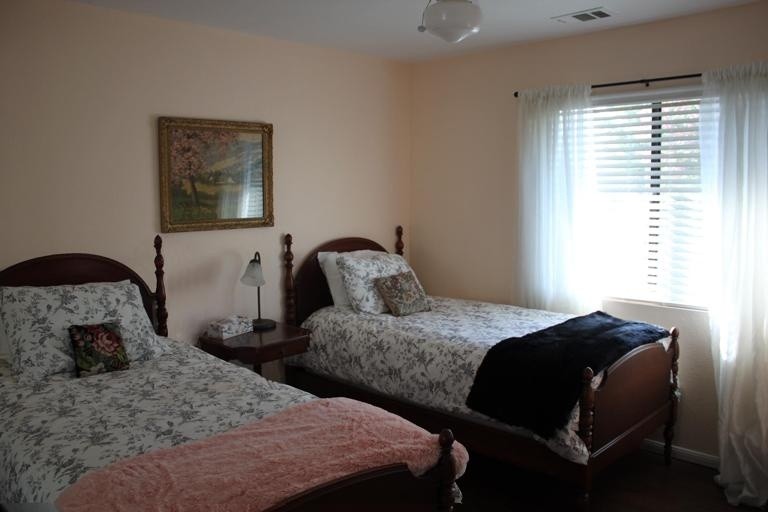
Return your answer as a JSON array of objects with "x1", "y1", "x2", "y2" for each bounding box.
[
  {"x1": 284, "y1": 225, "x2": 681, "y2": 504},
  {"x1": 0, "y1": 235, "x2": 456, "y2": 511}
]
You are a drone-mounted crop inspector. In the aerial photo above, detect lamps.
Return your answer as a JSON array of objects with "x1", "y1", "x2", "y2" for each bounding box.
[
  {"x1": 418, "y1": 0, "x2": 482, "y2": 45},
  {"x1": 240, "y1": 251, "x2": 276, "y2": 332}
]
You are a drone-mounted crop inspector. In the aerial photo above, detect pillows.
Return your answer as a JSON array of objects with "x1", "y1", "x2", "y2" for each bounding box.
[
  {"x1": 317, "y1": 249, "x2": 387, "y2": 310},
  {"x1": 374, "y1": 270, "x2": 432, "y2": 317},
  {"x1": 68, "y1": 322, "x2": 129, "y2": 377},
  {"x1": 336, "y1": 253, "x2": 425, "y2": 315}
]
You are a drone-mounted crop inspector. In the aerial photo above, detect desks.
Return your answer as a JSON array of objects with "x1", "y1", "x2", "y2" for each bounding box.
[{"x1": 198, "y1": 320, "x2": 311, "y2": 375}]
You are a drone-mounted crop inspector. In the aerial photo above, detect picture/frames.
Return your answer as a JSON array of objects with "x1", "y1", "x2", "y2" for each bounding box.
[{"x1": 158, "y1": 117, "x2": 274, "y2": 233}]
